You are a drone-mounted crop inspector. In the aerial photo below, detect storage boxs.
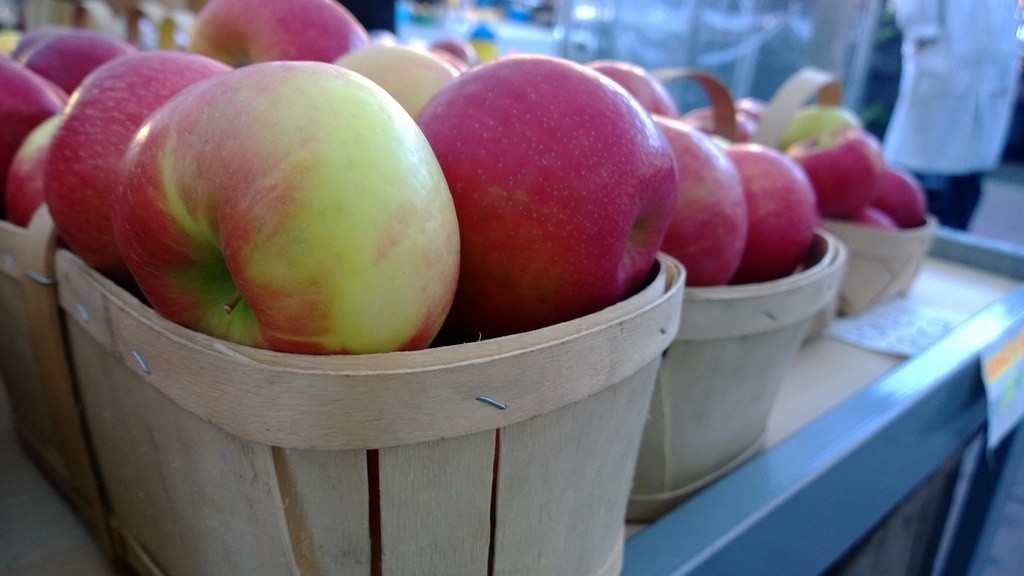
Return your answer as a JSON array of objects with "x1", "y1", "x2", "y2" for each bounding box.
[{"x1": 0, "y1": 69, "x2": 932, "y2": 576}]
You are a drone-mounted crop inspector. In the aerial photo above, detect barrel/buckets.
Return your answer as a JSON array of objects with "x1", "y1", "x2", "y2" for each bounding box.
[{"x1": 0, "y1": 64, "x2": 935, "y2": 575}]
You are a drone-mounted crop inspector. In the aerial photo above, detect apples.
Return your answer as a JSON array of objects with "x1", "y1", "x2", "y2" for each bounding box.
[{"x1": 2, "y1": 1, "x2": 926, "y2": 354}]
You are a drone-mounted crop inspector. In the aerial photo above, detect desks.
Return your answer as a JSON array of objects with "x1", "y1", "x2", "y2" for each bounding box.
[{"x1": 3, "y1": 213, "x2": 1024, "y2": 576}]
